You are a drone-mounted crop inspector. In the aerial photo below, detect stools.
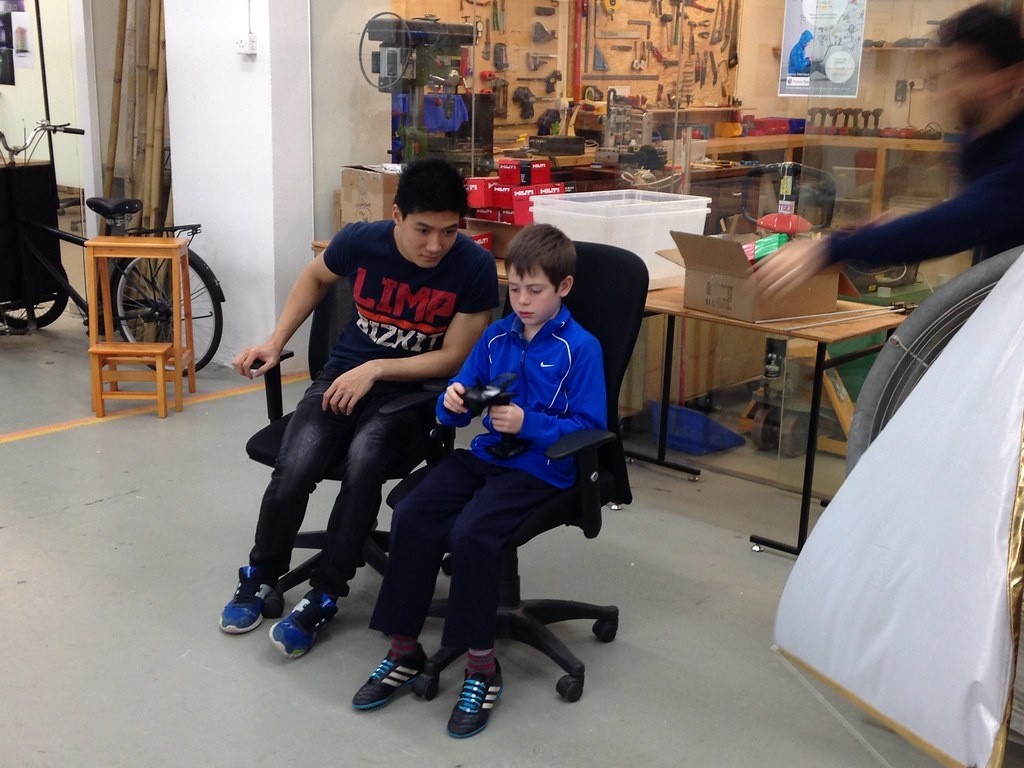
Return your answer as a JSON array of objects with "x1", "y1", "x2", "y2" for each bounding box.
[{"x1": 80, "y1": 235, "x2": 197, "y2": 419}]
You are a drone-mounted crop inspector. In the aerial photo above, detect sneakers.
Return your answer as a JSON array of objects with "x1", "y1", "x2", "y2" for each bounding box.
[
  {"x1": 446, "y1": 658, "x2": 502, "y2": 738},
  {"x1": 351, "y1": 642, "x2": 427, "y2": 709},
  {"x1": 219, "y1": 567, "x2": 279, "y2": 633},
  {"x1": 268, "y1": 590, "x2": 339, "y2": 657}
]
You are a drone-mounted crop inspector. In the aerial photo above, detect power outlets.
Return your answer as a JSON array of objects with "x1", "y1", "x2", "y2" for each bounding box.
[{"x1": 906, "y1": 78, "x2": 924, "y2": 89}]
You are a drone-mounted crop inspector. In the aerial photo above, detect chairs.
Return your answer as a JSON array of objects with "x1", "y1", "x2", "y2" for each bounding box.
[
  {"x1": 388, "y1": 240, "x2": 652, "y2": 707},
  {"x1": 245, "y1": 279, "x2": 456, "y2": 623}
]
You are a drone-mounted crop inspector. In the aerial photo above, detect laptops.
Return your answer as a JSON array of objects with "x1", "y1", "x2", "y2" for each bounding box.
[{"x1": 813, "y1": 47, "x2": 829, "y2": 63}]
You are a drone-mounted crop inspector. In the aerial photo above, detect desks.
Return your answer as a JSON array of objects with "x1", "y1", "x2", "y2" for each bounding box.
[
  {"x1": 706, "y1": 133, "x2": 960, "y2": 223},
  {"x1": 572, "y1": 161, "x2": 767, "y2": 234},
  {"x1": 632, "y1": 283, "x2": 908, "y2": 557},
  {"x1": 635, "y1": 106, "x2": 757, "y2": 113}
]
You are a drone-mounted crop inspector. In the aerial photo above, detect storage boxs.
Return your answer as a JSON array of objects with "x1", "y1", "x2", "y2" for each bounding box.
[
  {"x1": 492, "y1": 185, "x2": 514, "y2": 210},
  {"x1": 475, "y1": 208, "x2": 499, "y2": 221},
  {"x1": 714, "y1": 122, "x2": 743, "y2": 137},
  {"x1": 660, "y1": 123, "x2": 712, "y2": 140},
  {"x1": 529, "y1": 188, "x2": 713, "y2": 217},
  {"x1": 512, "y1": 181, "x2": 577, "y2": 227},
  {"x1": 341, "y1": 162, "x2": 402, "y2": 227},
  {"x1": 465, "y1": 177, "x2": 499, "y2": 208},
  {"x1": 656, "y1": 230, "x2": 861, "y2": 325},
  {"x1": 459, "y1": 229, "x2": 493, "y2": 252},
  {"x1": 788, "y1": 119, "x2": 806, "y2": 134},
  {"x1": 497, "y1": 159, "x2": 551, "y2": 185},
  {"x1": 754, "y1": 119, "x2": 780, "y2": 134},
  {"x1": 499, "y1": 210, "x2": 514, "y2": 223},
  {"x1": 527, "y1": 206, "x2": 712, "y2": 290},
  {"x1": 777, "y1": 119, "x2": 789, "y2": 134}
]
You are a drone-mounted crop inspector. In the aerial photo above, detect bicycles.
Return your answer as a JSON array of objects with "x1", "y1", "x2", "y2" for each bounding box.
[{"x1": 0, "y1": 120, "x2": 226, "y2": 377}]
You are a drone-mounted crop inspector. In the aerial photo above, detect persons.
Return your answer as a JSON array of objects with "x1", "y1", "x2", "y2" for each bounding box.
[
  {"x1": 220, "y1": 160, "x2": 499, "y2": 657},
  {"x1": 749, "y1": 0, "x2": 1024, "y2": 304},
  {"x1": 352, "y1": 223, "x2": 607, "y2": 739},
  {"x1": 787, "y1": 30, "x2": 826, "y2": 77}
]
